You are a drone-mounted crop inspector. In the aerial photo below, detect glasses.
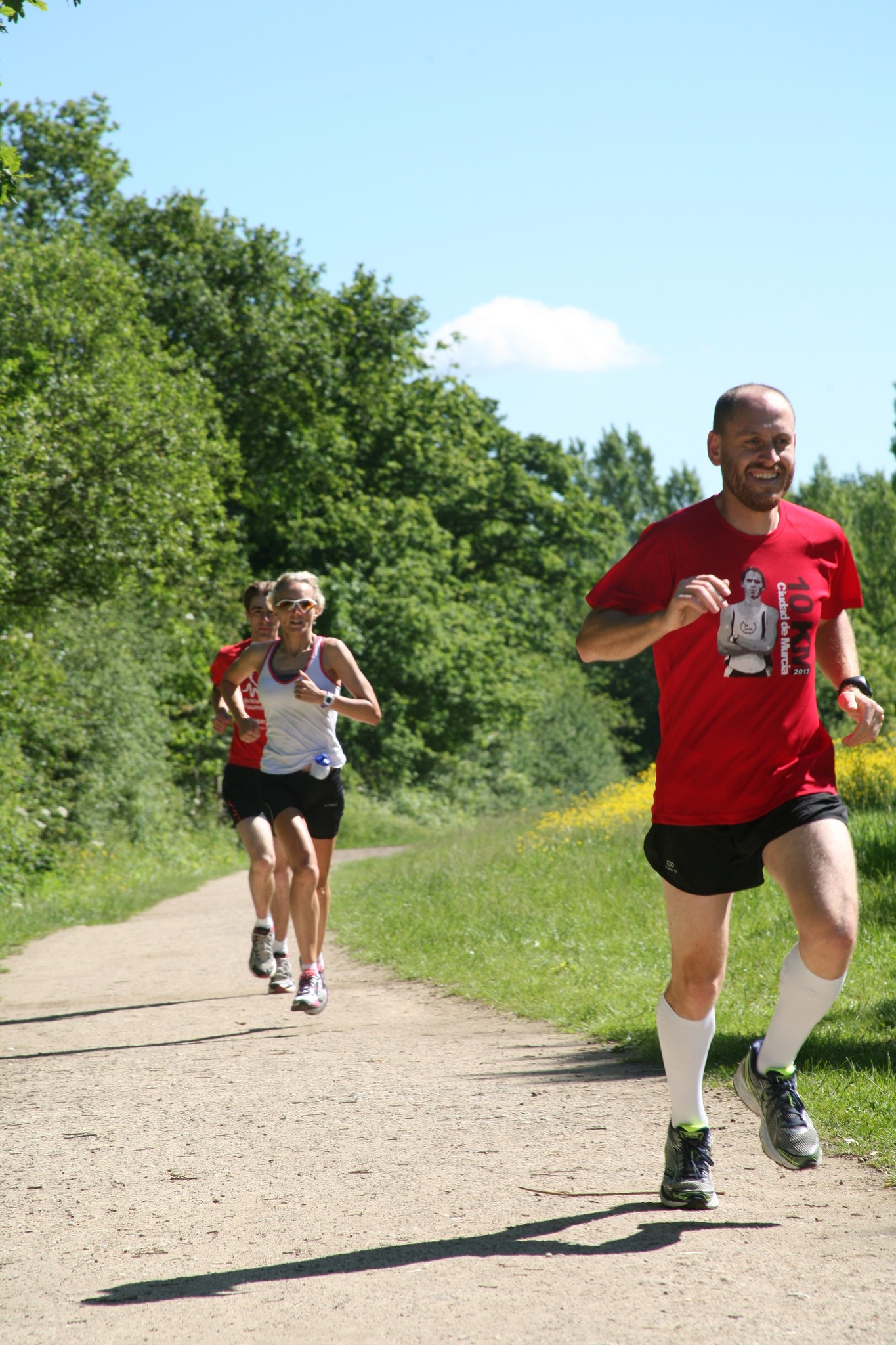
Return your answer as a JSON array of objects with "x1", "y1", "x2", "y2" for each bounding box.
[{"x1": 273, "y1": 598, "x2": 318, "y2": 611}]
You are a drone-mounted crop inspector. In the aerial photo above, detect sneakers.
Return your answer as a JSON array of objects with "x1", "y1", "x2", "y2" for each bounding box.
[
  {"x1": 659, "y1": 1115, "x2": 719, "y2": 1211},
  {"x1": 248, "y1": 915, "x2": 277, "y2": 978},
  {"x1": 268, "y1": 950, "x2": 296, "y2": 995},
  {"x1": 291, "y1": 955, "x2": 328, "y2": 1015},
  {"x1": 731, "y1": 1038, "x2": 824, "y2": 1171}
]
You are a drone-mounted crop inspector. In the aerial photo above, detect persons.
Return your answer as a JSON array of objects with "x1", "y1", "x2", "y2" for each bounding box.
[
  {"x1": 717, "y1": 567, "x2": 779, "y2": 678},
  {"x1": 225, "y1": 569, "x2": 386, "y2": 1018},
  {"x1": 566, "y1": 376, "x2": 890, "y2": 1207},
  {"x1": 210, "y1": 579, "x2": 312, "y2": 996}
]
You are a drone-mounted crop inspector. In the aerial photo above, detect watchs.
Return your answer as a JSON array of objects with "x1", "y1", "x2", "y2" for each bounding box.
[
  {"x1": 317, "y1": 690, "x2": 337, "y2": 711},
  {"x1": 836, "y1": 674, "x2": 875, "y2": 699},
  {"x1": 733, "y1": 633, "x2": 739, "y2": 642}
]
